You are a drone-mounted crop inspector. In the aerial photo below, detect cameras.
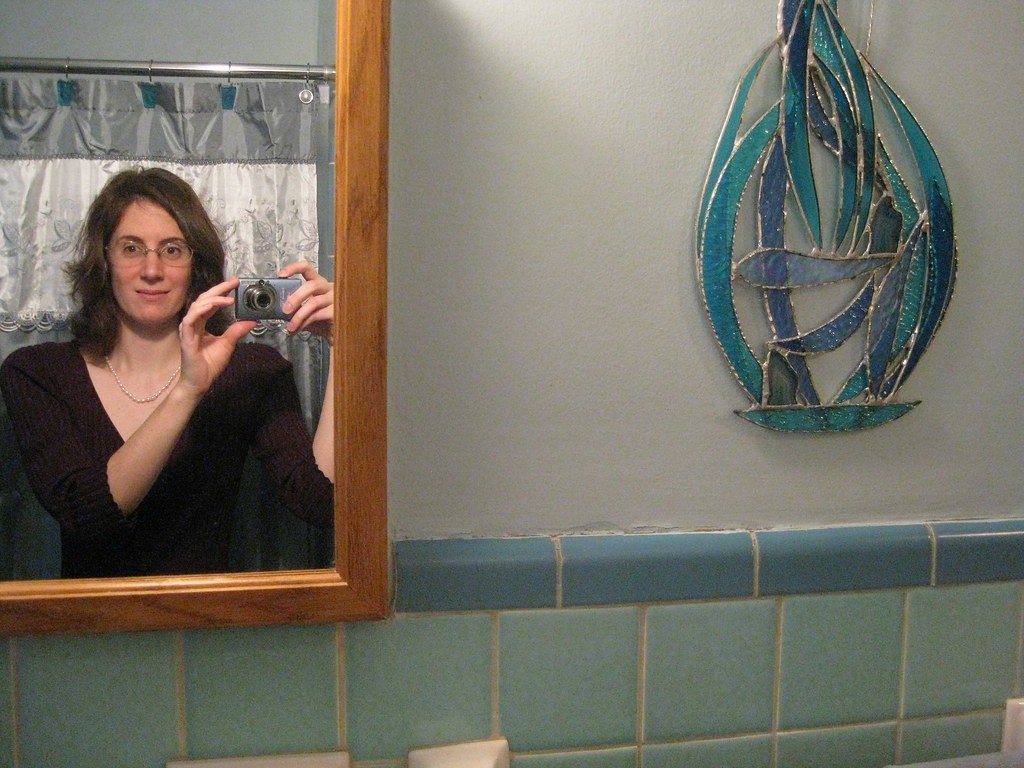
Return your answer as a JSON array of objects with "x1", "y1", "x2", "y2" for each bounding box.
[{"x1": 235, "y1": 277, "x2": 302, "y2": 320}]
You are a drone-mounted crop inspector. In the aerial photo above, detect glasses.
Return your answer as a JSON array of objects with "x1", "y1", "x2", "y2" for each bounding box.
[{"x1": 103, "y1": 241, "x2": 198, "y2": 266}]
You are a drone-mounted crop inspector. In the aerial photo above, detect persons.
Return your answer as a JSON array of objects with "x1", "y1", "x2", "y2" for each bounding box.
[{"x1": 0, "y1": 168, "x2": 334, "y2": 569}]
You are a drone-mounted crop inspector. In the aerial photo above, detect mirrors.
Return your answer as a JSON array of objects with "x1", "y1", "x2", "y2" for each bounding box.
[{"x1": 0, "y1": 0, "x2": 391, "y2": 638}]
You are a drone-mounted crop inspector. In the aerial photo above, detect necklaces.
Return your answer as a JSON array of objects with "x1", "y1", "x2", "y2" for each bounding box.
[{"x1": 104, "y1": 355, "x2": 181, "y2": 402}]
